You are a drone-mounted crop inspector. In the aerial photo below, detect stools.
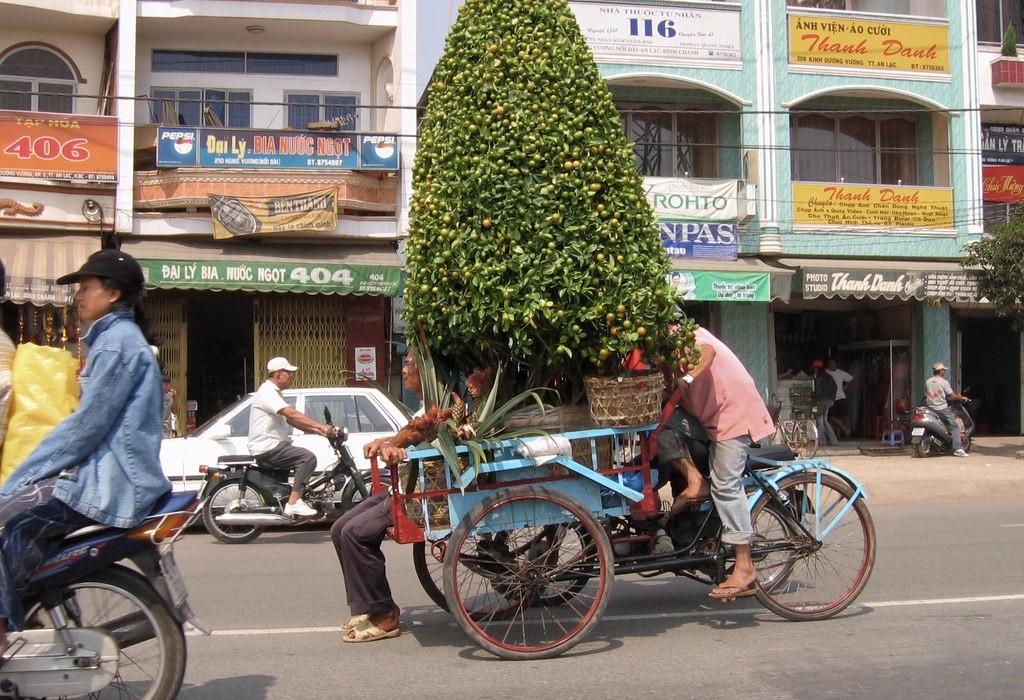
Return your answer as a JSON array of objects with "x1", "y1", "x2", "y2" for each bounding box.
[{"x1": 882, "y1": 430, "x2": 905, "y2": 447}]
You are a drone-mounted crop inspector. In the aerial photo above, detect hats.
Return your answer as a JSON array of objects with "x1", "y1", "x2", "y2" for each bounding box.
[
  {"x1": 812, "y1": 360, "x2": 823, "y2": 367},
  {"x1": 268, "y1": 357, "x2": 298, "y2": 374},
  {"x1": 56, "y1": 249, "x2": 145, "y2": 286},
  {"x1": 933, "y1": 362, "x2": 947, "y2": 371}
]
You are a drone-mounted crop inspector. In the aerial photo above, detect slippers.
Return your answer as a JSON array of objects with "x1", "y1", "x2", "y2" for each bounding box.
[
  {"x1": 342, "y1": 613, "x2": 371, "y2": 628},
  {"x1": 708, "y1": 582, "x2": 758, "y2": 598},
  {"x1": 671, "y1": 494, "x2": 712, "y2": 514},
  {"x1": 343, "y1": 619, "x2": 399, "y2": 642}
]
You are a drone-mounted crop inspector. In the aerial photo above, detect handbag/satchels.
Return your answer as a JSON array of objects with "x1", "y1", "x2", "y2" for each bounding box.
[{"x1": 813, "y1": 406, "x2": 825, "y2": 417}]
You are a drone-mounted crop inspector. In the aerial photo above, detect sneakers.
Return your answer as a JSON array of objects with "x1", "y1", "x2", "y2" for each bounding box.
[
  {"x1": 284, "y1": 498, "x2": 319, "y2": 516},
  {"x1": 954, "y1": 449, "x2": 969, "y2": 457}
]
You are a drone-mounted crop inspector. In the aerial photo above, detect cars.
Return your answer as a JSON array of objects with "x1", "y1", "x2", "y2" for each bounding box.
[{"x1": 159, "y1": 388, "x2": 418, "y2": 530}]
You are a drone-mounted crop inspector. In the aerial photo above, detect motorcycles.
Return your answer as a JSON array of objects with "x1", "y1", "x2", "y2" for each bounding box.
[{"x1": 198, "y1": 405, "x2": 394, "y2": 545}]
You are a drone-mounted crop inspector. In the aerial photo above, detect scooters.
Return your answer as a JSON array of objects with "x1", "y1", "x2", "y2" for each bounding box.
[
  {"x1": 909, "y1": 387, "x2": 975, "y2": 458},
  {"x1": 489, "y1": 485, "x2": 814, "y2": 607},
  {"x1": 1, "y1": 489, "x2": 212, "y2": 699}
]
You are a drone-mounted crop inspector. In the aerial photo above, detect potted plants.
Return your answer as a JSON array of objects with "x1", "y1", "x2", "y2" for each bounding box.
[{"x1": 400, "y1": 0, "x2": 702, "y2": 468}]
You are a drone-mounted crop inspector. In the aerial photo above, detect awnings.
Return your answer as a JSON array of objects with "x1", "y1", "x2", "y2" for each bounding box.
[
  {"x1": 120, "y1": 240, "x2": 406, "y2": 297},
  {"x1": 0, "y1": 234, "x2": 105, "y2": 308}
]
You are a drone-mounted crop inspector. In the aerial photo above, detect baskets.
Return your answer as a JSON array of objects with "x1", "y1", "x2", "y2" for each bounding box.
[{"x1": 766, "y1": 405, "x2": 778, "y2": 423}]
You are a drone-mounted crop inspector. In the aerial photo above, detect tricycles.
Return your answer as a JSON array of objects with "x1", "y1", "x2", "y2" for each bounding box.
[{"x1": 370, "y1": 422, "x2": 876, "y2": 662}]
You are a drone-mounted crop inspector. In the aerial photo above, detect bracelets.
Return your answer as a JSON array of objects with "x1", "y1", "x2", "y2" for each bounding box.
[{"x1": 683, "y1": 374, "x2": 694, "y2": 386}]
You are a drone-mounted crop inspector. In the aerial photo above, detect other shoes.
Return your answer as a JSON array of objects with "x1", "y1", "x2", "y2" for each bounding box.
[{"x1": 844, "y1": 428, "x2": 850, "y2": 439}]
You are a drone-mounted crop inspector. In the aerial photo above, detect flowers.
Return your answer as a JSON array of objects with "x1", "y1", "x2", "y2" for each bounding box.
[{"x1": 338, "y1": 316, "x2": 561, "y2": 497}]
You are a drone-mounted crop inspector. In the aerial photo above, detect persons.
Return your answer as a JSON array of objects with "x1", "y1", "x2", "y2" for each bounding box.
[
  {"x1": 250, "y1": 356, "x2": 334, "y2": 515},
  {"x1": 925, "y1": 362, "x2": 970, "y2": 456},
  {"x1": 0, "y1": 257, "x2": 178, "y2": 660},
  {"x1": 788, "y1": 359, "x2": 855, "y2": 447},
  {"x1": 649, "y1": 304, "x2": 776, "y2": 598},
  {"x1": 864, "y1": 352, "x2": 911, "y2": 438},
  {"x1": 331, "y1": 350, "x2": 427, "y2": 642}
]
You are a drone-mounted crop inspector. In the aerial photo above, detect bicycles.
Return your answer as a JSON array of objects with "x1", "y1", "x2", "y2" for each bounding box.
[{"x1": 764, "y1": 393, "x2": 819, "y2": 460}]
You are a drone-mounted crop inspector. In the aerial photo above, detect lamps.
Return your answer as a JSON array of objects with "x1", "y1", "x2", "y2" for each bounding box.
[{"x1": 246, "y1": 25, "x2": 264, "y2": 33}]
